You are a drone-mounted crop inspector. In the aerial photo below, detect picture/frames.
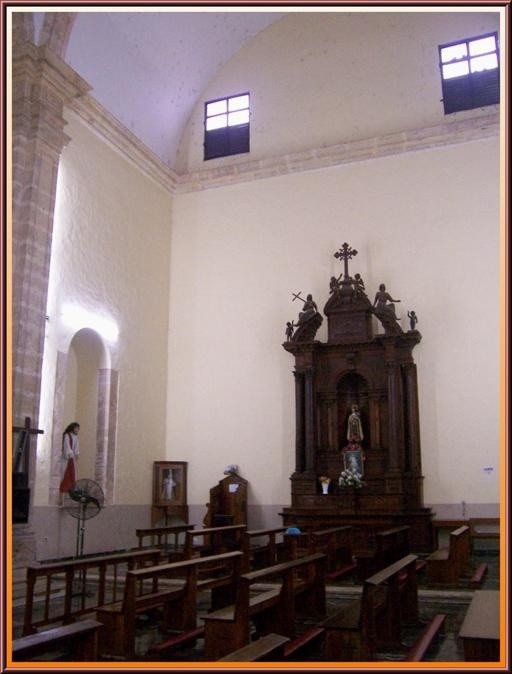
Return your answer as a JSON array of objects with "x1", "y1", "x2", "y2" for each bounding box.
[{"x1": 342, "y1": 449, "x2": 363, "y2": 472}]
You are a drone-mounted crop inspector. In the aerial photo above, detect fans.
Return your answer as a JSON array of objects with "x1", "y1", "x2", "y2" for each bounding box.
[{"x1": 63, "y1": 478, "x2": 105, "y2": 557}]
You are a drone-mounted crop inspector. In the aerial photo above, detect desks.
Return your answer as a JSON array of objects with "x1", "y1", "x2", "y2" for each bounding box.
[{"x1": 201, "y1": 475, "x2": 247, "y2": 529}]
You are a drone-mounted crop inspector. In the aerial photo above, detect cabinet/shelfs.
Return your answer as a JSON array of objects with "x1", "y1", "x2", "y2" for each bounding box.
[{"x1": 150, "y1": 460, "x2": 188, "y2": 524}]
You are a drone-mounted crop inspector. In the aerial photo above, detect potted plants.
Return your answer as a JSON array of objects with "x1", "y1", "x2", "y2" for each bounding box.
[{"x1": 318, "y1": 475, "x2": 330, "y2": 494}]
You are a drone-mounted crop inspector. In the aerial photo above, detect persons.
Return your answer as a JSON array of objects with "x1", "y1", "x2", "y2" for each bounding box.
[
  {"x1": 57, "y1": 422, "x2": 81, "y2": 510},
  {"x1": 285, "y1": 294, "x2": 317, "y2": 342},
  {"x1": 372, "y1": 284, "x2": 402, "y2": 321},
  {"x1": 349, "y1": 453, "x2": 358, "y2": 475},
  {"x1": 162, "y1": 474, "x2": 178, "y2": 502},
  {"x1": 346, "y1": 404, "x2": 364, "y2": 446},
  {"x1": 329, "y1": 273, "x2": 365, "y2": 301},
  {"x1": 407, "y1": 310, "x2": 418, "y2": 331}
]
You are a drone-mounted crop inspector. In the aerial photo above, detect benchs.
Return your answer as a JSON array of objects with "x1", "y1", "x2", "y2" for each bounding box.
[{"x1": 91, "y1": 523, "x2": 500, "y2": 663}]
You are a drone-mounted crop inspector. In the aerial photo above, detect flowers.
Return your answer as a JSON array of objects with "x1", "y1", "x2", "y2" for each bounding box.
[{"x1": 338, "y1": 468, "x2": 363, "y2": 490}]
